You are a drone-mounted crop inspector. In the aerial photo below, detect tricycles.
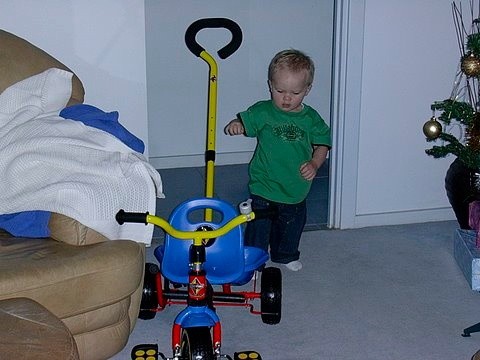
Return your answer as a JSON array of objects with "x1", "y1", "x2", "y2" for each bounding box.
[{"x1": 113, "y1": 17, "x2": 283, "y2": 360}]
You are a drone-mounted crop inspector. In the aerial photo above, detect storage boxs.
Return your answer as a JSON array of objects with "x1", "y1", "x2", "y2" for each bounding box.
[{"x1": 454, "y1": 201, "x2": 480, "y2": 290}]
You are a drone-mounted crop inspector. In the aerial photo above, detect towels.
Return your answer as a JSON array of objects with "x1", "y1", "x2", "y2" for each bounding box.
[{"x1": 0, "y1": 62, "x2": 165, "y2": 248}]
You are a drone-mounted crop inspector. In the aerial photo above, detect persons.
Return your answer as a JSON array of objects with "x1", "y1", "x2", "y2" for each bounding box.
[{"x1": 225, "y1": 48, "x2": 332, "y2": 281}]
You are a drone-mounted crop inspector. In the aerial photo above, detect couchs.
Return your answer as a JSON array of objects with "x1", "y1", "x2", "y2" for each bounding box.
[{"x1": 0, "y1": 30, "x2": 144, "y2": 360}]
